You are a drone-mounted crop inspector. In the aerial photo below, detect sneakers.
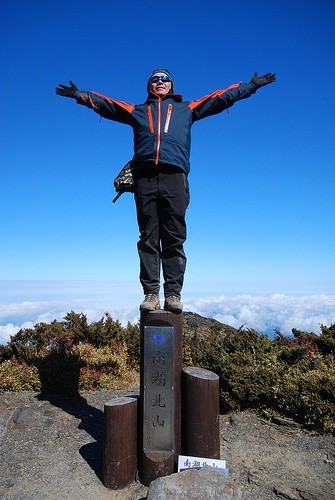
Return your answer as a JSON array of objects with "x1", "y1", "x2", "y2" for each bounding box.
[
  {"x1": 140, "y1": 293, "x2": 160, "y2": 311},
  {"x1": 164, "y1": 296, "x2": 183, "y2": 312}
]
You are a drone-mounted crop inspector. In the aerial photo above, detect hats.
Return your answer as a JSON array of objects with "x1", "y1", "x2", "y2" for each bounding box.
[{"x1": 148, "y1": 70, "x2": 173, "y2": 90}]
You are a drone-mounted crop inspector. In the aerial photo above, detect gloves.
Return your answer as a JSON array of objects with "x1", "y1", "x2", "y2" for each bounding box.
[
  {"x1": 252, "y1": 72, "x2": 277, "y2": 88},
  {"x1": 55, "y1": 80, "x2": 78, "y2": 98}
]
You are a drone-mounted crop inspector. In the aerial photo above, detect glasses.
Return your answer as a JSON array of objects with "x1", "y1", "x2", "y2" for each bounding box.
[{"x1": 151, "y1": 76, "x2": 170, "y2": 83}]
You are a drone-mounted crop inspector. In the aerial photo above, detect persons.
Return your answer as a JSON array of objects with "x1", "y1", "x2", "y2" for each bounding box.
[{"x1": 55, "y1": 69, "x2": 277, "y2": 313}]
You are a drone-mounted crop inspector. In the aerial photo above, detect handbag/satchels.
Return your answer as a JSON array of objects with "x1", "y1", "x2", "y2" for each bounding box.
[{"x1": 114, "y1": 160, "x2": 135, "y2": 191}]
